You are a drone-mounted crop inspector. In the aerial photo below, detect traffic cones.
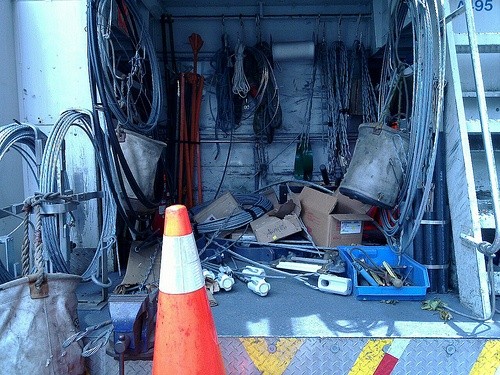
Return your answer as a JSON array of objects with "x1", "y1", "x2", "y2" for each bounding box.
[{"x1": 152, "y1": 205, "x2": 228, "y2": 375}]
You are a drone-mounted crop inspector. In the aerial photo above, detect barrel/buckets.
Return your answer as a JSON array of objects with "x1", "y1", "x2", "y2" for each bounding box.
[
  {"x1": 1, "y1": 273, "x2": 87, "y2": 375},
  {"x1": 110, "y1": 128, "x2": 167, "y2": 200},
  {"x1": 340, "y1": 122, "x2": 410, "y2": 210}
]
